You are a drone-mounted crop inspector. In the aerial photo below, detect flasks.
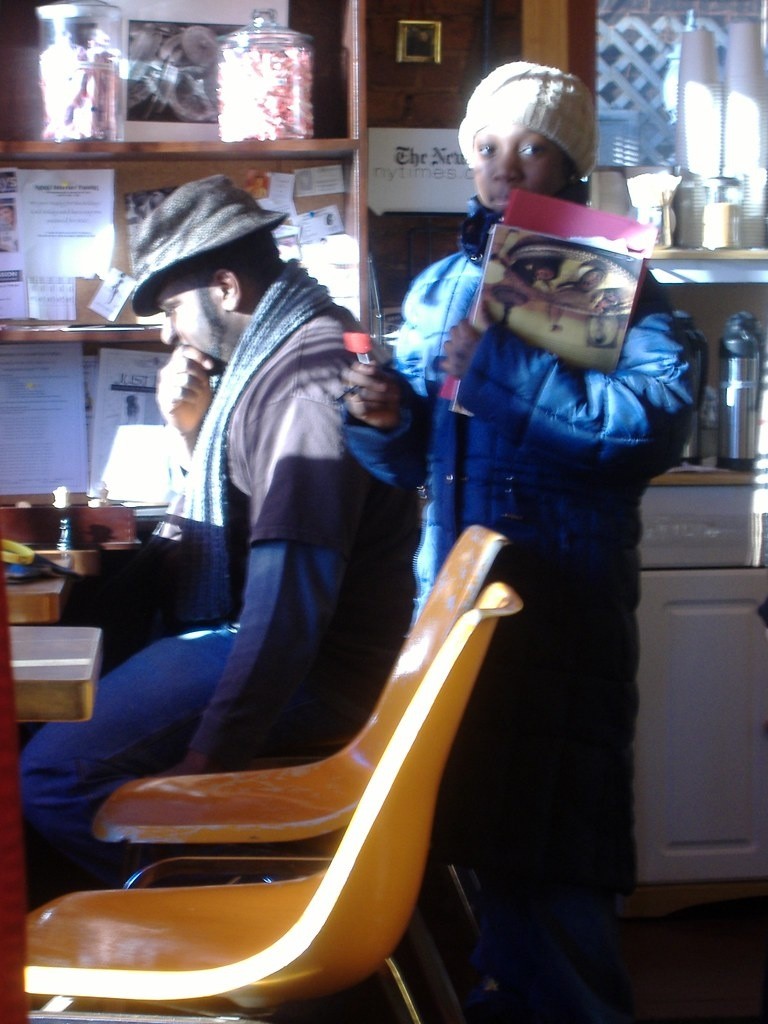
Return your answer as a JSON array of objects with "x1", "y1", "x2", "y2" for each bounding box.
[{"x1": 719, "y1": 313, "x2": 763, "y2": 473}]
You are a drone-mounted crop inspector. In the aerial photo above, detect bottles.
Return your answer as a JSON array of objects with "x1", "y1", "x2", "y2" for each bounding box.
[
  {"x1": 36, "y1": 0, "x2": 123, "y2": 141},
  {"x1": 660, "y1": 308, "x2": 708, "y2": 463},
  {"x1": 56, "y1": 517, "x2": 72, "y2": 551},
  {"x1": 701, "y1": 176, "x2": 740, "y2": 250},
  {"x1": 217, "y1": 7, "x2": 315, "y2": 141}
]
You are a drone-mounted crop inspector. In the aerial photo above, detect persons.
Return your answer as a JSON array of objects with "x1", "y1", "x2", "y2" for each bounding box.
[
  {"x1": 0, "y1": 174, "x2": 422, "y2": 889},
  {"x1": 336, "y1": 61, "x2": 706, "y2": 1024}
]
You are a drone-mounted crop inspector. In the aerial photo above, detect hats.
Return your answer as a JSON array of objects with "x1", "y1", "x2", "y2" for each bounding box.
[
  {"x1": 457, "y1": 60, "x2": 597, "y2": 180},
  {"x1": 129, "y1": 173, "x2": 289, "y2": 317}
]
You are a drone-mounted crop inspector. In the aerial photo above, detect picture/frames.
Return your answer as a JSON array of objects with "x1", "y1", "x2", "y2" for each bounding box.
[{"x1": 395, "y1": 19, "x2": 441, "y2": 64}]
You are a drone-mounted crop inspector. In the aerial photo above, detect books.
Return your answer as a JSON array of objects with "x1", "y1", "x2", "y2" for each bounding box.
[{"x1": 440, "y1": 187, "x2": 657, "y2": 414}]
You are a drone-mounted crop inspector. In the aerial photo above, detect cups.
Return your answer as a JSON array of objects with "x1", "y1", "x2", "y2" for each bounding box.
[{"x1": 675, "y1": 19, "x2": 767, "y2": 249}]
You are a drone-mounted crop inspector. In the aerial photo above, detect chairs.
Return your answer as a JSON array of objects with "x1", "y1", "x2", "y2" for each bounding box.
[
  {"x1": 24, "y1": 579, "x2": 526, "y2": 1024},
  {"x1": 92, "y1": 526, "x2": 512, "y2": 1024}
]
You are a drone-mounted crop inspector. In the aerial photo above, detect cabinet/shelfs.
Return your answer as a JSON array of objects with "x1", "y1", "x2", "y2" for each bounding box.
[
  {"x1": 611, "y1": 1, "x2": 765, "y2": 924},
  {"x1": 0, "y1": 0, "x2": 597, "y2": 501}
]
